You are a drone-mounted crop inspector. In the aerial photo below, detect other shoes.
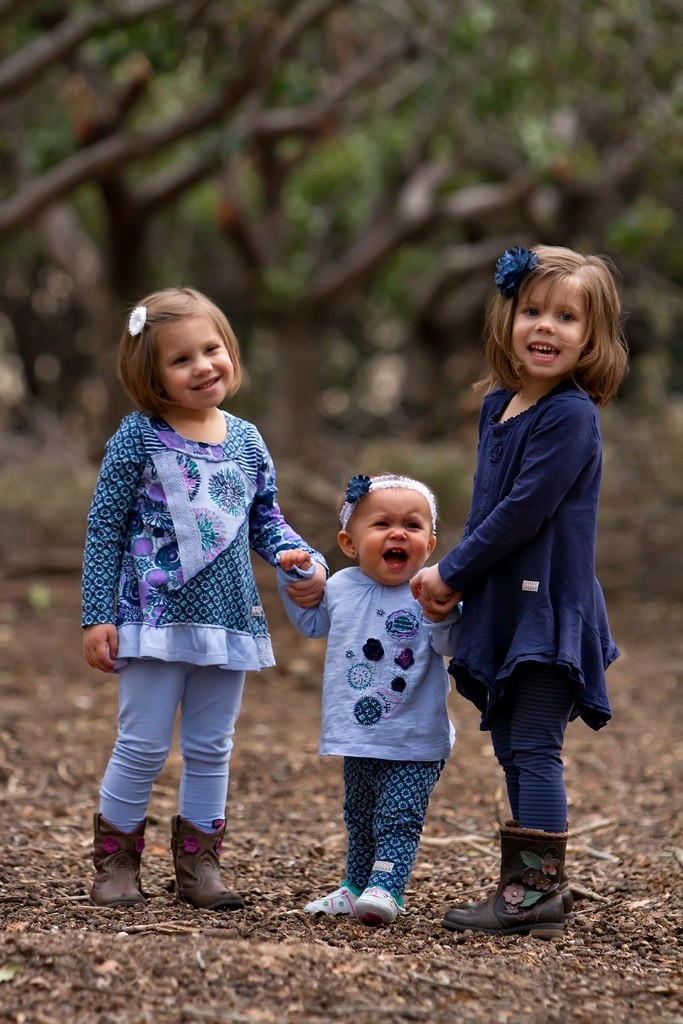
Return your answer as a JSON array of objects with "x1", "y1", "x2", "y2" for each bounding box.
[
  {"x1": 301, "y1": 881, "x2": 362, "y2": 917},
  {"x1": 353, "y1": 883, "x2": 404, "y2": 928}
]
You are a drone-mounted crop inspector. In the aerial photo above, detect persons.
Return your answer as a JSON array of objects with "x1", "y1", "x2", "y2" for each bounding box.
[
  {"x1": 412, "y1": 241, "x2": 630, "y2": 939},
  {"x1": 81, "y1": 286, "x2": 332, "y2": 914},
  {"x1": 274, "y1": 469, "x2": 464, "y2": 927}
]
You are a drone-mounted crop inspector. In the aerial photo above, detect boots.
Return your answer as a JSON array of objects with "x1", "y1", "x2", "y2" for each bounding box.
[
  {"x1": 87, "y1": 812, "x2": 150, "y2": 907},
  {"x1": 441, "y1": 821, "x2": 569, "y2": 940},
  {"x1": 453, "y1": 820, "x2": 576, "y2": 919},
  {"x1": 169, "y1": 814, "x2": 244, "y2": 910}
]
